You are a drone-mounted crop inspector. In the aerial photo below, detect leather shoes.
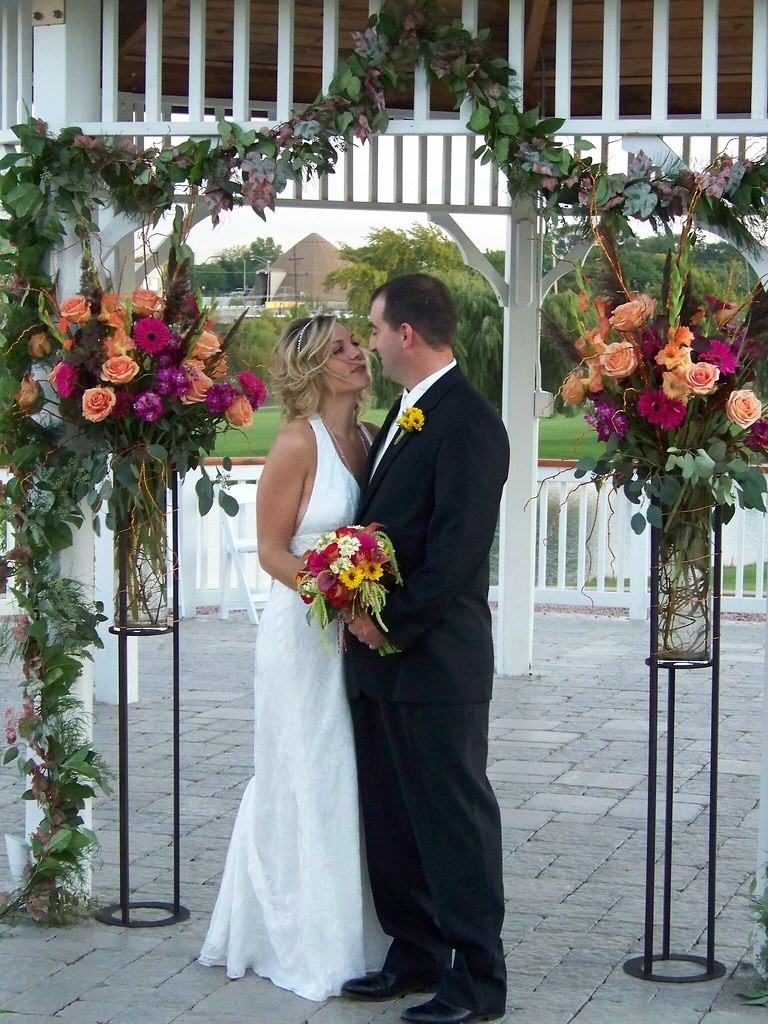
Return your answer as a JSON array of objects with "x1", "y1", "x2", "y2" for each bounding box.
[
  {"x1": 341, "y1": 970, "x2": 437, "y2": 1002},
  {"x1": 399, "y1": 995, "x2": 504, "y2": 1024}
]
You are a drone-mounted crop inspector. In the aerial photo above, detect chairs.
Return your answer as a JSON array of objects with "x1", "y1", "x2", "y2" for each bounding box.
[{"x1": 216, "y1": 477, "x2": 277, "y2": 627}]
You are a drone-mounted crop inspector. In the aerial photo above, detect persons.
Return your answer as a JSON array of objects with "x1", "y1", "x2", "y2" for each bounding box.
[{"x1": 192, "y1": 274, "x2": 512, "y2": 1024}]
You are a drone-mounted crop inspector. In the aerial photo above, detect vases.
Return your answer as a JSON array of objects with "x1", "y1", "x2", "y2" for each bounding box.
[
  {"x1": 654, "y1": 499, "x2": 714, "y2": 664},
  {"x1": 114, "y1": 451, "x2": 168, "y2": 630}
]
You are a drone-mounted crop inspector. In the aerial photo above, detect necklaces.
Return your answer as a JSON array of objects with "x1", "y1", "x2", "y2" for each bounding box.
[{"x1": 323, "y1": 419, "x2": 369, "y2": 476}]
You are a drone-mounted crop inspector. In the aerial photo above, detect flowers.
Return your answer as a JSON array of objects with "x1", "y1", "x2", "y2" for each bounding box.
[
  {"x1": 394, "y1": 408, "x2": 425, "y2": 446},
  {"x1": 296, "y1": 522, "x2": 404, "y2": 656},
  {"x1": 557, "y1": 149, "x2": 768, "y2": 657},
  {"x1": 0, "y1": 2, "x2": 768, "y2": 1008},
  {"x1": 15, "y1": 204, "x2": 267, "y2": 625}
]
